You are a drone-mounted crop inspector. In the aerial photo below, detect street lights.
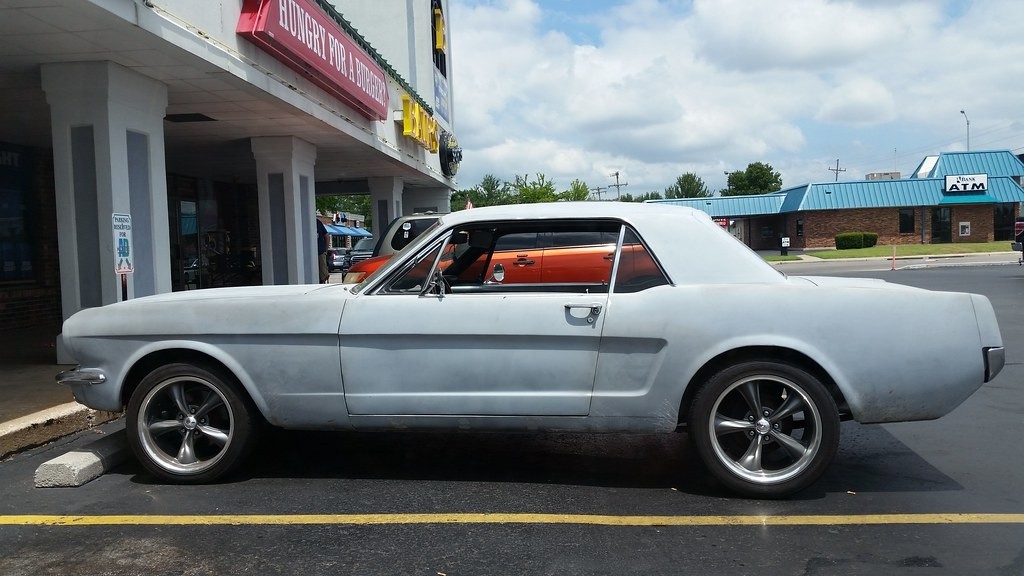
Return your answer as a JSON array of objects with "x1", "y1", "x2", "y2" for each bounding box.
[{"x1": 961, "y1": 110, "x2": 970, "y2": 151}]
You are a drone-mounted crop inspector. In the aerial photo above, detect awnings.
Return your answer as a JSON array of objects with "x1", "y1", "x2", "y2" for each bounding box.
[{"x1": 323, "y1": 224, "x2": 373, "y2": 236}]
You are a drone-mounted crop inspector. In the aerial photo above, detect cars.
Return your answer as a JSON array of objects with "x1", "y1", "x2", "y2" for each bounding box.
[
  {"x1": 56, "y1": 200, "x2": 1005, "y2": 498},
  {"x1": 1011, "y1": 216, "x2": 1024, "y2": 266},
  {"x1": 174, "y1": 258, "x2": 209, "y2": 283}
]
